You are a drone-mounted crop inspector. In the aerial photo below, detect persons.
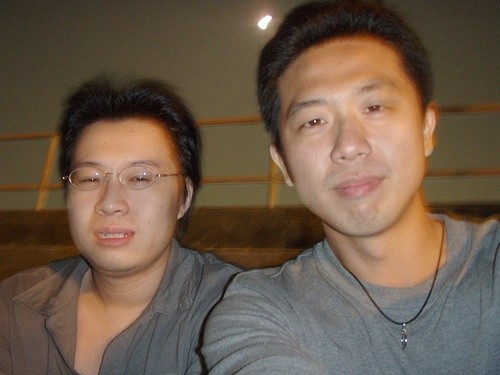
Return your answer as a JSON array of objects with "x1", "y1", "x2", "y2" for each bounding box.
[
  {"x1": 0, "y1": 69, "x2": 246, "y2": 375},
  {"x1": 197, "y1": 0, "x2": 500, "y2": 375}
]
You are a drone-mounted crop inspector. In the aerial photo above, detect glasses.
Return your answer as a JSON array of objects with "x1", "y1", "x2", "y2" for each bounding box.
[{"x1": 62, "y1": 166, "x2": 181, "y2": 192}]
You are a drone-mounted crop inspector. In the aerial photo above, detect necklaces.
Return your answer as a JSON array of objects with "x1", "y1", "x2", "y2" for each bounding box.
[{"x1": 336, "y1": 220, "x2": 447, "y2": 352}]
